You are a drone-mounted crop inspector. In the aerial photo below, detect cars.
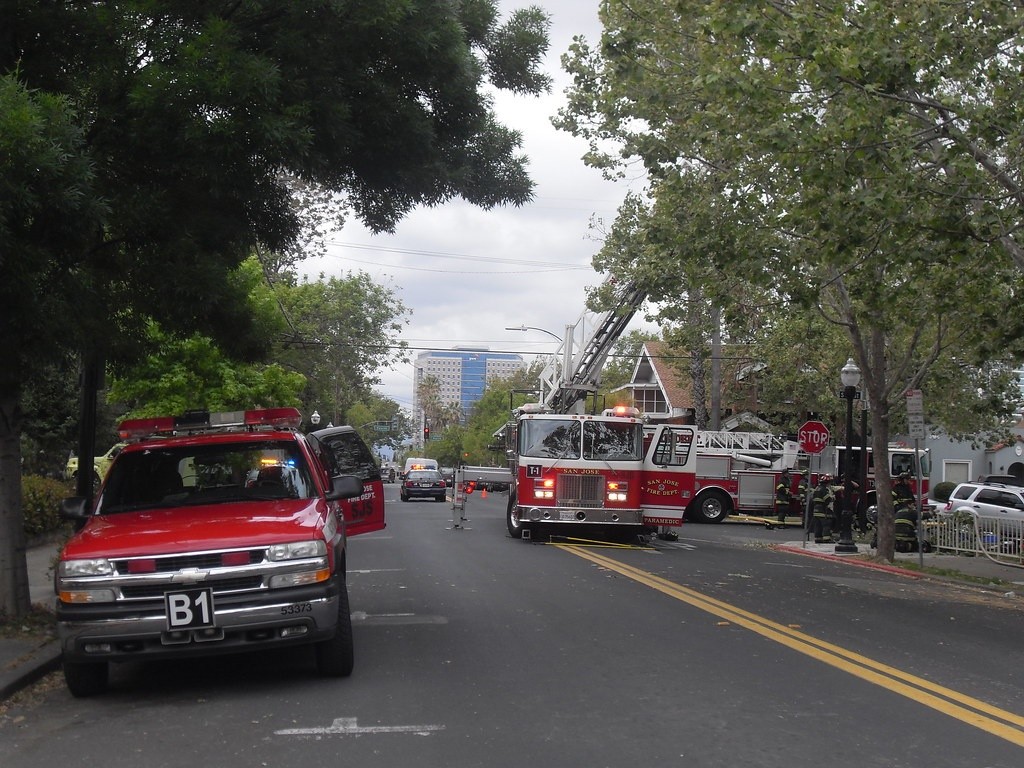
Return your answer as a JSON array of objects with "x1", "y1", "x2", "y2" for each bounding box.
[{"x1": 397, "y1": 464, "x2": 446, "y2": 502}]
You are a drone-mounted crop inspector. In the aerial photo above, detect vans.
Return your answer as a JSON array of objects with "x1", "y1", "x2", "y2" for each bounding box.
[
  {"x1": 380, "y1": 467, "x2": 395, "y2": 483},
  {"x1": 405, "y1": 458, "x2": 439, "y2": 474}
]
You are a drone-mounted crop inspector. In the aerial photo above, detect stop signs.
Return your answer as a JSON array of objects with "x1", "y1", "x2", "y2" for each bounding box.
[{"x1": 797, "y1": 420, "x2": 830, "y2": 454}]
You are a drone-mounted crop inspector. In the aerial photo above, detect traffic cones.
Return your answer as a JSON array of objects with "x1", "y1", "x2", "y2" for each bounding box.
[{"x1": 480, "y1": 488, "x2": 488, "y2": 499}]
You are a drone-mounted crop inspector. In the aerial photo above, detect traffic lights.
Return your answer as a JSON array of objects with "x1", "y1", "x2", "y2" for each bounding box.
[{"x1": 424, "y1": 428, "x2": 429, "y2": 439}]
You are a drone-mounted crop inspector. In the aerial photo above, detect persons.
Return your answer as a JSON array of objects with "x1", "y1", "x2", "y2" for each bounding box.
[
  {"x1": 799, "y1": 470, "x2": 923, "y2": 553},
  {"x1": 776, "y1": 469, "x2": 793, "y2": 529}
]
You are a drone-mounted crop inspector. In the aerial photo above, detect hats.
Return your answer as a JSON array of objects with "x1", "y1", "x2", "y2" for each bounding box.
[{"x1": 782, "y1": 469, "x2": 789, "y2": 474}]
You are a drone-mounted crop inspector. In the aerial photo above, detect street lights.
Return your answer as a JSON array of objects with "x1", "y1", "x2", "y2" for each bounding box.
[
  {"x1": 441, "y1": 406, "x2": 466, "y2": 430},
  {"x1": 310, "y1": 410, "x2": 320, "y2": 432},
  {"x1": 833, "y1": 358, "x2": 862, "y2": 554}
]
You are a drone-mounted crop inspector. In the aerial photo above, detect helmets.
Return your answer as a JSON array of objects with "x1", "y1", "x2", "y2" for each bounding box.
[
  {"x1": 800, "y1": 469, "x2": 809, "y2": 478},
  {"x1": 819, "y1": 476, "x2": 831, "y2": 484},
  {"x1": 899, "y1": 472, "x2": 910, "y2": 481},
  {"x1": 922, "y1": 539, "x2": 932, "y2": 553}
]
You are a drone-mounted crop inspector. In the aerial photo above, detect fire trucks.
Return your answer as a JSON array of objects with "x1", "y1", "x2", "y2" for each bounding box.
[
  {"x1": 644, "y1": 423, "x2": 932, "y2": 524},
  {"x1": 445, "y1": 236, "x2": 699, "y2": 543}
]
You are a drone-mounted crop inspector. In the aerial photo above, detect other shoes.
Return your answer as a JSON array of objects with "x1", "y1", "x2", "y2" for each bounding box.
[
  {"x1": 815, "y1": 539, "x2": 822, "y2": 543},
  {"x1": 784, "y1": 525, "x2": 790, "y2": 529},
  {"x1": 779, "y1": 525, "x2": 786, "y2": 529},
  {"x1": 822, "y1": 538, "x2": 836, "y2": 544}
]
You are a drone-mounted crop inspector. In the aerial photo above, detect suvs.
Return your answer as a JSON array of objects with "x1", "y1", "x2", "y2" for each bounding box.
[
  {"x1": 52, "y1": 406, "x2": 387, "y2": 695},
  {"x1": 928, "y1": 482, "x2": 1024, "y2": 539},
  {"x1": 65, "y1": 442, "x2": 196, "y2": 498}
]
